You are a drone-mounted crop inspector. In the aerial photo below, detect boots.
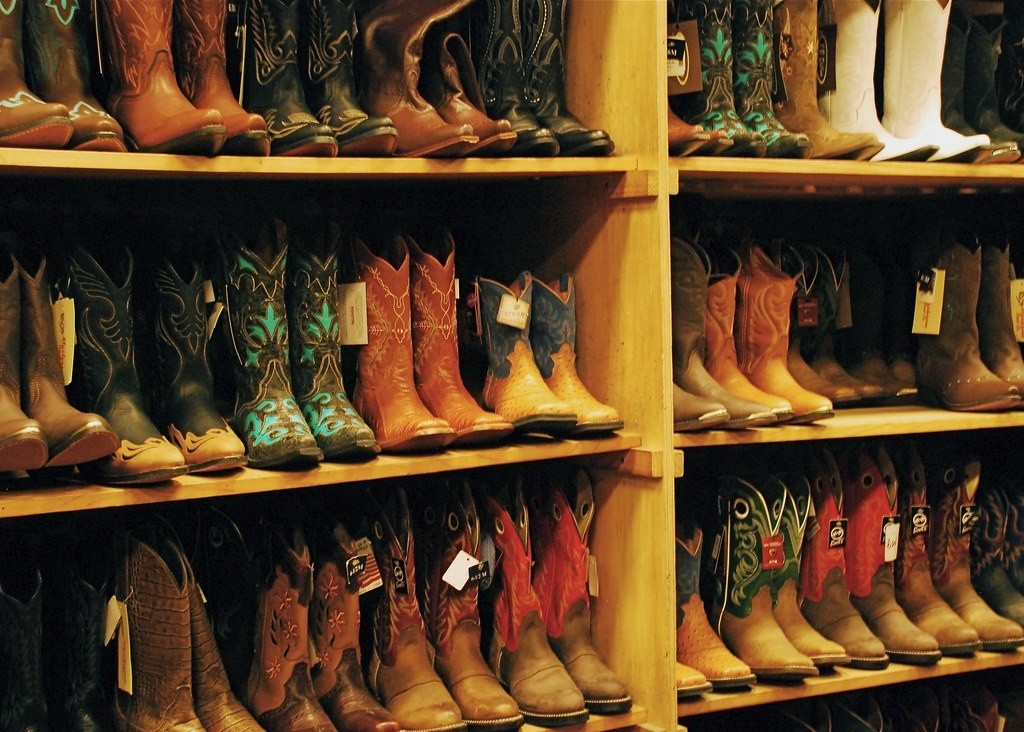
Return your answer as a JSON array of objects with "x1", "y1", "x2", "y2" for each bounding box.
[{"x1": 0, "y1": 0, "x2": 1024, "y2": 732}]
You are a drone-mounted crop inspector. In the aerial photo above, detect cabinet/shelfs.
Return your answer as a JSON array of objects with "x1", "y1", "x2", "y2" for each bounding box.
[{"x1": 0, "y1": 0, "x2": 1023, "y2": 732}]
